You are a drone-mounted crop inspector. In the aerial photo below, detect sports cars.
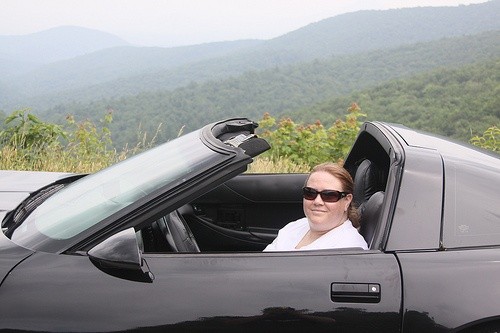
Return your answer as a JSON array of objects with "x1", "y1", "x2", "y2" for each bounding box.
[{"x1": 0, "y1": 117, "x2": 500, "y2": 333}]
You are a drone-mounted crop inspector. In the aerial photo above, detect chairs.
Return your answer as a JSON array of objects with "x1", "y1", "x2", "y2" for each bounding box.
[
  {"x1": 358, "y1": 191, "x2": 384, "y2": 248},
  {"x1": 352, "y1": 158, "x2": 374, "y2": 209}
]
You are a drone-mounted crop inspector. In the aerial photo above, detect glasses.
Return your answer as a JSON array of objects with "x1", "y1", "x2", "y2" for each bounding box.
[{"x1": 301, "y1": 187, "x2": 348, "y2": 203}]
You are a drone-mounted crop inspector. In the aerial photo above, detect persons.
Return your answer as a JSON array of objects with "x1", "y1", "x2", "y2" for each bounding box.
[{"x1": 261, "y1": 161, "x2": 370, "y2": 250}]
